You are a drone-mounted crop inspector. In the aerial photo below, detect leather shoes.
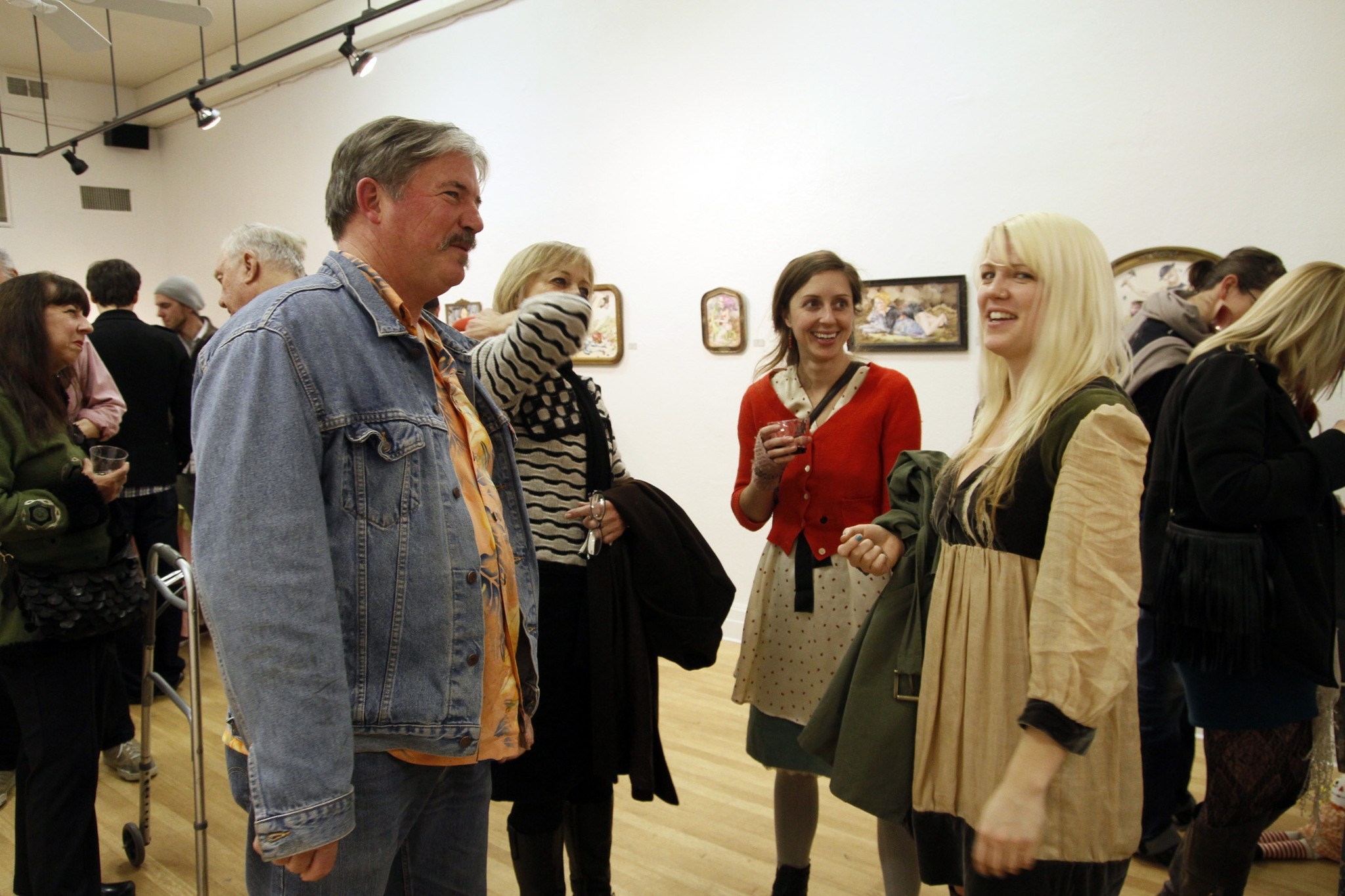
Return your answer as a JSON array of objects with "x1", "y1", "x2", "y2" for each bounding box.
[{"x1": 100, "y1": 881, "x2": 135, "y2": 896}]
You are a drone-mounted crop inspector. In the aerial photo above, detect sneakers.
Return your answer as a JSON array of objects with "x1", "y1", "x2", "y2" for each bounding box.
[
  {"x1": 102, "y1": 738, "x2": 157, "y2": 782},
  {"x1": 0, "y1": 770, "x2": 17, "y2": 806}
]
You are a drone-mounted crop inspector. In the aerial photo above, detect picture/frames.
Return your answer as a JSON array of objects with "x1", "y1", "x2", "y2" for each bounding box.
[
  {"x1": 701, "y1": 287, "x2": 745, "y2": 354},
  {"x1": 570, "y1": 284, "x2": 623, "y2": 364},
  {"x1": 847, "y1": 275, "x2": 967, "y2": 351},
  {"x1": 1111, "y1": 247, "x2": 1225, "y2": 325}
]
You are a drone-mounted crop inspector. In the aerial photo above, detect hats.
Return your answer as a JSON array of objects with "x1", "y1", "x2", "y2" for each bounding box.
[{"x1": 156, "y1": 277, "x2": 206, "y2": 311}]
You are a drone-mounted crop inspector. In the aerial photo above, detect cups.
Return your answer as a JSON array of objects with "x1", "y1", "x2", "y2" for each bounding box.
[
  {"x1": 766, "y1": 418, "x2": 810, "y2": 455},
  {"x1": 89, "y1": 445, "x2": 129, "y2": 475}
]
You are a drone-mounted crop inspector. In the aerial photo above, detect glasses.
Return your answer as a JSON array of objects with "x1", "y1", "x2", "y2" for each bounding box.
[{"x1": 578, "y1": 490, "x2": 605, "y2": 560}]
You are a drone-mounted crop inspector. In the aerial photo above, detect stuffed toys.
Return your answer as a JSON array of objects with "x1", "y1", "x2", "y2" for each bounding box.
[{"x1": 1254, "y1": 775, "x2": 1345, "y2": 862}]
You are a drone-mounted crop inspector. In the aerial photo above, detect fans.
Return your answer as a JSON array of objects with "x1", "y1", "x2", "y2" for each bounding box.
[{"x1": 6, "y1": 0, "x2": 212, "y2": 53}]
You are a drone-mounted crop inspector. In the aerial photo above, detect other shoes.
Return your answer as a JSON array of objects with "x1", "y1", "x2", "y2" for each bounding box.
[{"x1": 1142, "y1": 834, "x2": 1181, "y2": 865}]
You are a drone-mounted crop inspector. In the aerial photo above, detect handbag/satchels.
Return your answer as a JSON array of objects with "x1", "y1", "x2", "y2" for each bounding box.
[{"x1": 7, "y1": 535, "x2": 150, "y2": 640}]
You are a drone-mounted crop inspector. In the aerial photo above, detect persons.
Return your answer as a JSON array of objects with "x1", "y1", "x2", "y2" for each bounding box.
[
  {"x1": 836, "y1": 213, "x2": 1152, "y2": 896},
  {"x1": 154, "y1": 280, "x2": 221, "y2": 645},
  {"x1": 0, "y1": 272, "x2": 149, "y2": 896},
  {"x1": 1135, "y1": 260, "x2": 1345, "y2": 896},
  {"x1": 213, "y1": 224, "x2": 311, "y2": 315},
  {"x1": 0, "y1": 252, "x2": 158, "y2": 811},
  {"x1": 190, "y1": 118, "x2": 540, "y2": 896},
  {"x1": 1123, "y1": 247, "x2": 1287, "y2": 871},
  {"x1": 85, "y1": 260, "x2": 196, "y2": 703},
  {"x1": 451, "y1": 241, "x2": 633, "y2": 896},
  {"x1": 730, "y1": 249, "x2": 922, "y2": 896}
]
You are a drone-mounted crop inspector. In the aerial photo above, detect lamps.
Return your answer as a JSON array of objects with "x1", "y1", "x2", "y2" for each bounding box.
[
  {"x1": 186, "y1": 92, "x2": 221, "y2": 132},
  {"x1": 62, "y1": 141, "x2": 88, "y2": 176},
  {"x1": 338, "y1": 27, "x2": 377, "y2": 79}
]
